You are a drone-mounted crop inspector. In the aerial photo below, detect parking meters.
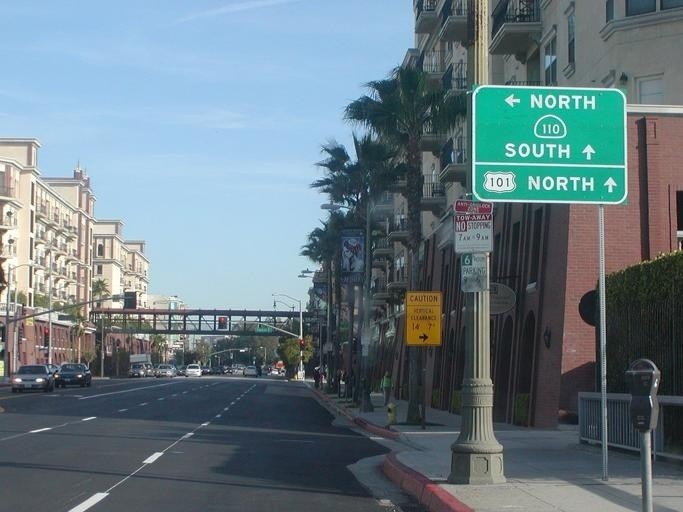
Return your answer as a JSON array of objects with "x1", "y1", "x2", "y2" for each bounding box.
[{"x1": 622, "y1": 353, "x2": 662, "y2": 512}]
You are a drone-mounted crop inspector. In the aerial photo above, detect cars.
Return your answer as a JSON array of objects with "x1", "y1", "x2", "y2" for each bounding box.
[
  {"x1": 126, "y1": 360, "x2": 271, "y2": 381},
  {"x1": 10, "y1": 361, "x2": 93, "y2": 395}
]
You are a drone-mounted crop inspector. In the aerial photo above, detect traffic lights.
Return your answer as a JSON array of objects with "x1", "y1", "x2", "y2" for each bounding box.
[
  {"x1": 217, "y1": 316, "x2": 227, "y2": 329},
  {"x1": 43, "y1": 327, "x2": 49, "y2": 348}
]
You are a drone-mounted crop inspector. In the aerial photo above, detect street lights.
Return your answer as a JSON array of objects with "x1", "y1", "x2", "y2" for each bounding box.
[
  {"x1": 247, "y1": 346, "x2": 266, "y2": 364},
  {"x1": 0, "y1": 262, "x2": 39, "y2": 388},
  {"x1": 46, "y1": 284, "x2": 72, "y2": 365},
  {"x1": 319, "y1": 203, "x2": 375, "y2": 414},
  {"x1": 97, "y1": 313, "x2": 106, "y2": 378},
  {"x1": 271, "y1": 268, "x2": 326, "y2": 389}
]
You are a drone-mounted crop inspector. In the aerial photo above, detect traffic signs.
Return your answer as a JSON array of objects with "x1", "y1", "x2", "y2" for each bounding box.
[
  {"x1": 404, "y1": 290, "x2": 444, "y2": 348},
  {"x1": 254, "y1": 326, "x2": 272, "y2": 334},
  {"x1": 469, "y1": 79, "x2": 631, "y2": 208}
]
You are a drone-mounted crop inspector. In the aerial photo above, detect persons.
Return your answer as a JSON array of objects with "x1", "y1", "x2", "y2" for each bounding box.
[
  {"x1": 379, "y1": 370, "x2": 394, "y2": 407},
  {"x1": 313, "y1": 370, "x2": 321, "y2": 391}
]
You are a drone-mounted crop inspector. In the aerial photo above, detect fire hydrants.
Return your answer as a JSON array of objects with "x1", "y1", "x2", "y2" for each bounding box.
[{"x1": 385, "y1": 400, "x2": 398, "y2": 427}]
